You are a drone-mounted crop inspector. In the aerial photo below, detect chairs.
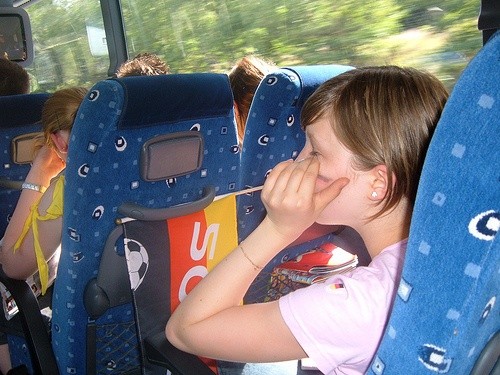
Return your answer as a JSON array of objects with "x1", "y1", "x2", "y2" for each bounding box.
[
  {"x1": 235, "y1": 65, "x2": 358, "y2": 305},
  {"x1": 0, "y1": 73, "x2": 242, "y2": 375},
  {"x1": 0, "y1": 92, "x2": 56, "y2": 242},
  {"x1": 361, "y1": 29, "x2": 500, "y2": 375}
]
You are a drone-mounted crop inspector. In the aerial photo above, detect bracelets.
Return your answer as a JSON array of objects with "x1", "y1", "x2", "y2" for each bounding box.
[
  {"x1": 22, "y1": 182, "x2": 47, "y2": 193},
  {"x1": 239, "y1": 242, "x2": 263, "y2": 271}
]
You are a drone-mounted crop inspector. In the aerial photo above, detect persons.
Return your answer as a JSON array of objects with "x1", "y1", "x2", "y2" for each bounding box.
[
  {"x1": 165, "y1": 66, "x2": 449, "y2": 375},
  {"x1": 0, "y1": 56, "x2": 280, "y2": 375}
]
